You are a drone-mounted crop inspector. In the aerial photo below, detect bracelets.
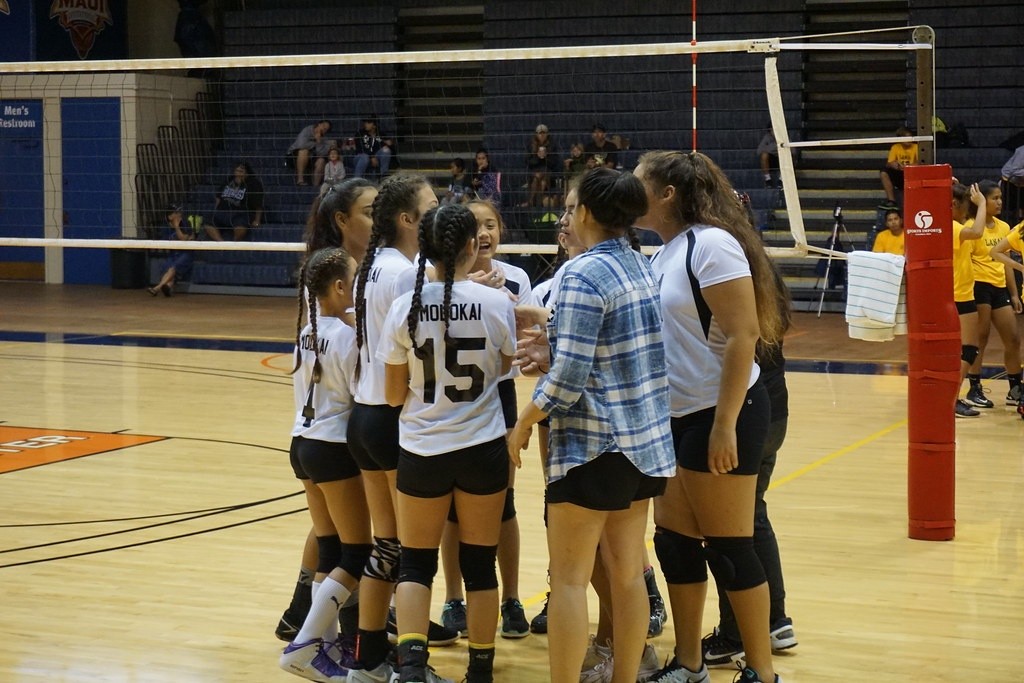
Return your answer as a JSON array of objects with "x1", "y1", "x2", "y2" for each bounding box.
[{"x1": 538, "y1": 365, "x2": 548, "y2": 374}]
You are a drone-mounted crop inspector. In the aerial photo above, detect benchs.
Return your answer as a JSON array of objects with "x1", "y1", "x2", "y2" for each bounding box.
[{"x1": 142, "y1": 0, "x2": 1024, "y2": 312}]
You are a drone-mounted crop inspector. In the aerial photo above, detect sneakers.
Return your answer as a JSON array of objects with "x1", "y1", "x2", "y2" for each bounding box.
[
  {"x1": 531, "y1": 592, "x2": 550, "y2": 633},
  {"x1": 346, "y1": 659, "x2": 402, "y2": 683},
  {"x1": 965, "y1": 385, "x2": 993, "y2": 407},
  {"x1": 393, "y1": 653, "x2": 449, "y2": 683},
  {"x1": 580, "y1": 638, "x2": 614, "y2": 683},
  {"x1": 274, "y1": 609, "x2": 303, "y2": 642},
  {"x1": 387, "y1": 606, "x2": 462, "y2": 646},
  {"x1": 279, "y1": 637, "x2": 349, "y2": 683},
  {"x1": 324, "y1": 633, "x2": 362, "y2": 671},
  {"x1": 440, "y1": 598, "x2": 468, "y2": 637},
  {"x1": 647, "y1": 598, "x2": 667, "y2": 638},
  {"x1": 636, "y1": 654, "x2": 710, "y2": 683},
  {"x1": 955, "y1": 399, "x2": 980, "y2": 418},
  {"x1": 501, "y1": 598, "x2": 530, "y2": 637},
  {"x1": 702, "y1": 626, "x2": 746, "y2": 670},
  {"x1": 637, "y1": 644, "x2": 659, "y2": 683},
  {"x1": 733, "y1": 666, "x2": 779, "y2": 683},
  {"x1": 770, "y1": 617, "x2": 798, "y2": 651}
]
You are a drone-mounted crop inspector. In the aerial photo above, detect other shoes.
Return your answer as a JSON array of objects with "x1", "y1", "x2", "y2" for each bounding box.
[{"x1": 1006, "y1": 391, "x2": 1019, "y2": 405}]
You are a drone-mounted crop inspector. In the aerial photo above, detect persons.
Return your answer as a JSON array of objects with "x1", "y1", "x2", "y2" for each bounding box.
[
  {"x1": 146, "y1": 204, "x2": 196, "y2": 296},
  {"x1": 989, "y1": 219, "x2": 1024, "y2": 417},
  {"x1": 288, "y1": 121, "x2": 330, "y2": 186},
  {"x1": 203, "y1": 163, "x2": 265, "y2": 241},
  {"x1": 274, "y1": 166, "x2": 676, "y2": 683},
  {"x1": 1001, "y1": 146, "x2": 1024, "y2": 228},
  {"x1": 352, "y1": 118, "x2": 392, "y2": 180},
  {"x1": 950, "y1": 176, "x2": 986, "y2": 417},
  {"x1": 517, "y1": 124, "x2": 560, "y2": 207},
  {"x1": 441, "y1": 150, "x2": 500, "y2": 206},
  {"x1": 756, "y1": 123, "x2": 795, "y2": 187},
  {"x1": 633, "y1": 151, "x2": 779, "y2": 683},
  {"x1": 321, "y1": 149, "x2": 346, "y2": 193},
  {"x1": 878, "y1": 129, "x2": 919, "y2": 210},
  {"x1": 965, "y1": 181, "x2": 1024, "y2": 407},
  {"x1": 873, "y1": 209, "x2": 904, "y2": 255},
  {"x1": 561, "y1": 126, "x2": 618, "y2": 205},
  {"x1": 673, "y1": 190, "x2": 798, "y2": 667}
]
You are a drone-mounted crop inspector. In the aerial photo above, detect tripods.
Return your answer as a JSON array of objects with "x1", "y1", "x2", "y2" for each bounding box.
[{"x1": 807, "y1": 215, "x2": 855, "y2": 318}]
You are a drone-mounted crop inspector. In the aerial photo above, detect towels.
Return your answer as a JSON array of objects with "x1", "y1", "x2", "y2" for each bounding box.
[{"x1": 845, "y1": 248, "x2": 910, "y2": 343}]
